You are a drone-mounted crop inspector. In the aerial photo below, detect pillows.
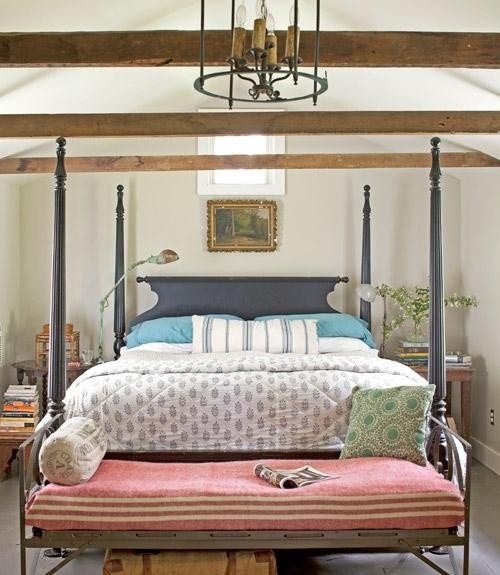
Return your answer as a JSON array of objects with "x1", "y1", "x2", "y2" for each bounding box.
[
  {"x1": 342, "y1": 386, "x2": 436, "y2": 468},
  {"x1": 121, "y1": 306, "x2": 375, "y2": 357},
  {"x1": 37, "y1": 417, "x2": 108, "y2": 486}
]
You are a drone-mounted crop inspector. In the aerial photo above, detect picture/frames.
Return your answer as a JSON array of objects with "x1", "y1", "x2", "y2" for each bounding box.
[{"x1": 205, "y1": 200, "x2": 280, "y2": 252}]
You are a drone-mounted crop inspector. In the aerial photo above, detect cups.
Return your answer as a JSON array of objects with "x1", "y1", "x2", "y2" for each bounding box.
[{"x1": 82, "y1": 349, "x2": 93, "y2": 363}]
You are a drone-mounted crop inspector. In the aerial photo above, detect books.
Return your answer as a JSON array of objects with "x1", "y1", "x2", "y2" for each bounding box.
[
  {"x1": 1, "y1": 384, "x2": 43, "y2": 442},
  {"x1": 253, "y1": 463, "x2": 339, "y2": 489},
  {"x1": 392, "y1": 339, "x2": 430, "y2": 366},
  {"x1": 446, "y1": 351, "x2": 473, "y2": 369}
]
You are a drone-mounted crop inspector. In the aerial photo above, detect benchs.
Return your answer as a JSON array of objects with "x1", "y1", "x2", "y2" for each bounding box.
[{"x1": 14, "y1": 412, "x2": 472, "y2": 574}]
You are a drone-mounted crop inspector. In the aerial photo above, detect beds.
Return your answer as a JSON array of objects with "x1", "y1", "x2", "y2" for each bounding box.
[{"x1": 18, "y1": 136, "x2": 444, "y2": 460}]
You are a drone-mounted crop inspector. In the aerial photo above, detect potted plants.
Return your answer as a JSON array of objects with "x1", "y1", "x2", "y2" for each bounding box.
[{"x1": 370, "y1": 285, "x2": 480, "y2": 344}]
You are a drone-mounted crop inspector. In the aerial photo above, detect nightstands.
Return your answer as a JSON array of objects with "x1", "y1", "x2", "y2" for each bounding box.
[
  {"x1": 411, "y1": 367, "x2": 473, "y2": 442},
  {"x1": 12, "y1": 360, "x2": 97, "y2": 416}
]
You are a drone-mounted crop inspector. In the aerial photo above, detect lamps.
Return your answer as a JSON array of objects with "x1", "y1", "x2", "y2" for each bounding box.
[
  {"x1": 94, "y1": 250, "x2": 180, "y2": 361},
  {"x1": 355, "y1": 282, "x2": 390, "y2": 357},
  {"x1": 189, "y1": 0, "x2": 328, "y2": 107}
]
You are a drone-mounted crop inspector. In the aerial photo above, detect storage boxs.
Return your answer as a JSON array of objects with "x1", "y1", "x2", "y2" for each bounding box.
[{"x1": 99, "y1": 548, "x2": 278, "y2": 575}]
